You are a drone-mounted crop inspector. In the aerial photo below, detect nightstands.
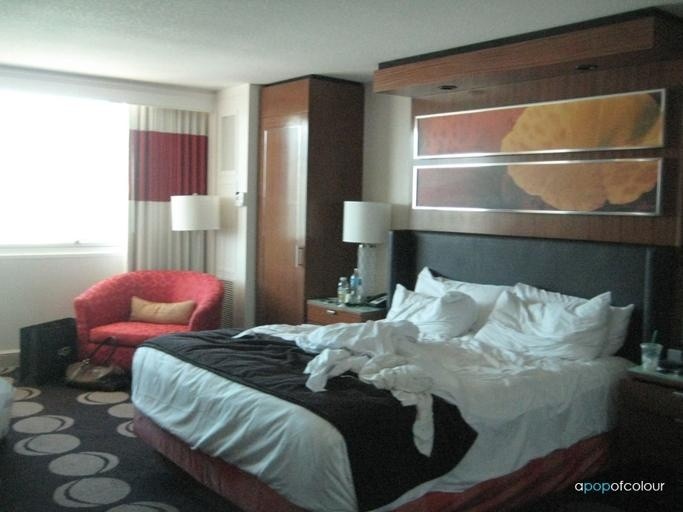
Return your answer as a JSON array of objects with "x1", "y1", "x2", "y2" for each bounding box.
[
  {"x1": 307, "y1": 294, "x2": 386, "y2": 325},
  {"x1": 620, "y1": 361, "x2": 681, "y2": 476}
]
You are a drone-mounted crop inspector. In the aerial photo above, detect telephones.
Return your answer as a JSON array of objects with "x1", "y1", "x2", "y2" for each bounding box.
[{"x1": 358, "y1": 292, "x2": 388, "y2": 308}]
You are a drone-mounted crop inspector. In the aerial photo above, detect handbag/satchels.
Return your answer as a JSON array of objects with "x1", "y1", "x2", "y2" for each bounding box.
[
  {"x1": 65, "y1": 336, "x2": 130, "y2": 391},
  {"x1": 20, "y1": 317, "x2": 76, "y2": 385}
]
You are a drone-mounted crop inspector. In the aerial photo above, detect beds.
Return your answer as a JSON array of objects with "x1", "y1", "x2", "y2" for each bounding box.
[{"x1": 129, "y1": 227, "x2": 657, "y2": 511}]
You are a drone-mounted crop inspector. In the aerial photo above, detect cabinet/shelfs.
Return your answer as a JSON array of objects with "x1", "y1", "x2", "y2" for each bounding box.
[{"x1": 253, "y1": 75, "x2": 362, "y2": 326}]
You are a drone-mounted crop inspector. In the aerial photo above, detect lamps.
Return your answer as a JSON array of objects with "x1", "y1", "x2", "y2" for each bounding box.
[
  {"x1": 341, "y1": 200, "x2": 388, "y2": 279},
  {"x1": 169, "y1": 192, "x2": 220, "y2": 272}
]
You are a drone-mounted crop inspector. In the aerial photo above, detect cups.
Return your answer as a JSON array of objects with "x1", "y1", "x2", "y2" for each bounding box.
[{"x1": 640, "y1": 343, "x2": 663, "y2": 373}]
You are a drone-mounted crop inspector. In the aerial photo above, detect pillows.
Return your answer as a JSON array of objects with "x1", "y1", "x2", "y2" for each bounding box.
[
  {"x1": 410, "y1": 263, "x2": 501, "y2": 333},
  {"x1": 515, "y1": 278, "x2": 633, "y2": 360},
  {"x1": 381, "y1": 282, "x2": 478, "y2": 342},
  {"x1": 128, "y1": 294, "x2": 196, "y2": 325},
  {"x1": 472, "y1": 291, "x2": 614, "y2": 365}
]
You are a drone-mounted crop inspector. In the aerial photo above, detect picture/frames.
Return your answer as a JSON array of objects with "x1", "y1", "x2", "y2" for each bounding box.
[
  {"x1": 411, "y1": 87, "x2": 670, "y2": 161},
  {"x1": 406, "y1": 156, "x2": 665, "y2": 218}
]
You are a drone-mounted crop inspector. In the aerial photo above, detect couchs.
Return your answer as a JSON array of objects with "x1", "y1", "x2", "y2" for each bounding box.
[{"x1": 74, "y1": 270, "x2": 225, "y2": 370}]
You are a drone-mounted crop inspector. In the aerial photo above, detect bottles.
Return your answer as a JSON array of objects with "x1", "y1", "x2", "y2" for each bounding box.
[
  {"x1": 349, "y1": 268, "x2": 362, "y2": 305},
  {"x1": 337, "y1": 277, "x2": 350, "y2": 304}
]
